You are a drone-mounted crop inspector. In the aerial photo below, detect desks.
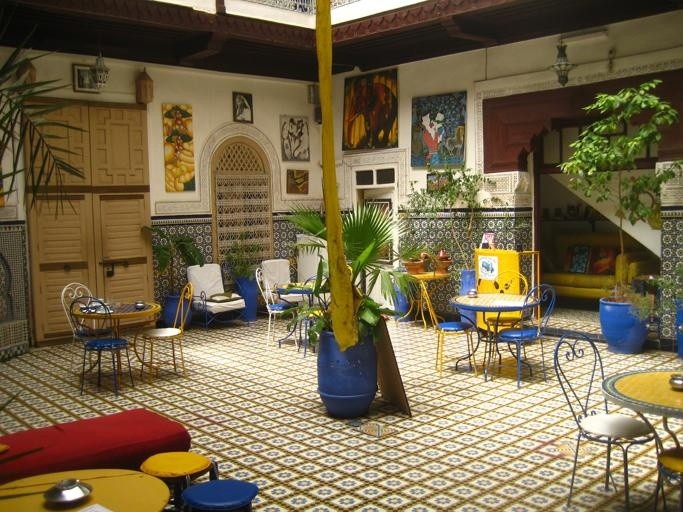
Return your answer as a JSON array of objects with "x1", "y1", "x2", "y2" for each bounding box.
[
  {"x1": 601, "y1": 369, "x2": 681, "y2": 512},
  {"x1": 0, "y1": 467, "x2": 172, "y2": 512}
]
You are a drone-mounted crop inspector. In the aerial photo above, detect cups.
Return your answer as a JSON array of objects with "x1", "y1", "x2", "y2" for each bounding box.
[
  {"x1": 516, "y1": 243, "x2": 523, "y2": 252},
  {"x1": 98, "y1": 298, "x2": 109, "y2": 311},
  {"x1": 490, "y1": 242, "x2": 497, "y2": 249},
  {"x1": 482, "y1": 242, "x2": 489, "y2": 249}
]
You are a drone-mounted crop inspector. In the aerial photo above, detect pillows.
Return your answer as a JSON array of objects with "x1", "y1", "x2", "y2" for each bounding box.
[
  {"x1": 590, "y1": 245, "x2": 617, "y2": 275},
  {"x1": 564, "y1": 243, "x2": 591, "y2": 274}
]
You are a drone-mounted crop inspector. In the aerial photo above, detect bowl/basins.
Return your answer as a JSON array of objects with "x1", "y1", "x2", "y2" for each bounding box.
[
  {"x1": 134, "y1": 300, "x2": 145, "y2": 309},
  {"x1": 43, "y1": 477, "x2": 92, "y2": 503},
  {"x1": 467, "y1": 288, "x2": 477, "y2": 298},
  {"x1": 669, "y1": 374, "x2": 682, "y2": 390}
]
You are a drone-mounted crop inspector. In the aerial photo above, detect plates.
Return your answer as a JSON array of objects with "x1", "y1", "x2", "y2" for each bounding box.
[{"x1": 96, "y1": 310, "x2": 114, "y2": 314}]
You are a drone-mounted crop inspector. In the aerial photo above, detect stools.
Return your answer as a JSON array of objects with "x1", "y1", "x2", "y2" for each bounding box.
[
  {"x1": 178, "y1": 477, "x2": 259, "y2": 511},
  {"x1": 137, "y1": 449, "x2": 221, "y2": 487}
]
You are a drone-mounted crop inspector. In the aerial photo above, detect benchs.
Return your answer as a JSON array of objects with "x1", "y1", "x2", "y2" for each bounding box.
[{"x1": 0, "y1": 410, "x2": 191, "y2": 471}]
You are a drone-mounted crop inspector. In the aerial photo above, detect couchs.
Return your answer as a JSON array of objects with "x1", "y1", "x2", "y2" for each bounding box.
[{"x1": 540, "y1": 230, "x2": 659, "y2": 299}]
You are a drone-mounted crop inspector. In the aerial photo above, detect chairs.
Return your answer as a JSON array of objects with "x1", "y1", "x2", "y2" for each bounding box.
[
  {"x1": 57, "y1": 258, "x2": 324, "y2": 401},
  {"x1": 256, "y1": 259, "x2": 331, "y2": 357},
  {"x1": 416, "y1": 271, "x2": 555, "y2": 389},
  {"x1": 556, "y1": 332, "x2": 664, "y2": 512}
]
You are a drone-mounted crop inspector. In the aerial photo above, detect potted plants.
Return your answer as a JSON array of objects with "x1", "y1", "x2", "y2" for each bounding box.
[
  {"x1": 222, "y1": 231, "x2": 262, "y2": 322},
  {"x1": 555, "y1": 75, "x2": 683, "y2": 357},
  {"x1": 278, "y1": 201, "x2": 430, "y2": 420},
  {"x1": 139, "y1": 224, "x2": 205, "y2": 330}
]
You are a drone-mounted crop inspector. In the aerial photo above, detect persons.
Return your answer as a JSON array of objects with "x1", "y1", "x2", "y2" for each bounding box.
[
  {"x1": 422, "y1": 111, "x2": 439, "y2": 165},
  {"x1": 432, "y1": 113, "x2": 446, "y2": 140}
]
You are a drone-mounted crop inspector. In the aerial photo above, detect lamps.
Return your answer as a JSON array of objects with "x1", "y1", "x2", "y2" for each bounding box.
[
  {"x1": 547, "y1": 32, "x2": 578, "y2": 85},
  {"x1": 87, "y1": 51, "x2": 111, "y2": 90}
]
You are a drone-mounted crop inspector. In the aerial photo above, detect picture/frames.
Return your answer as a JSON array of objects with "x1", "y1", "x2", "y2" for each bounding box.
[
  {"x1": 71, "y1": 63, "x2": 102, "y2": 93},
  {"x1": 232, "y1": 90, "x2": 253, "y2": 123},
  {"x1": 426, "y1": 171, "x2": 452, "y2": 195}
]
